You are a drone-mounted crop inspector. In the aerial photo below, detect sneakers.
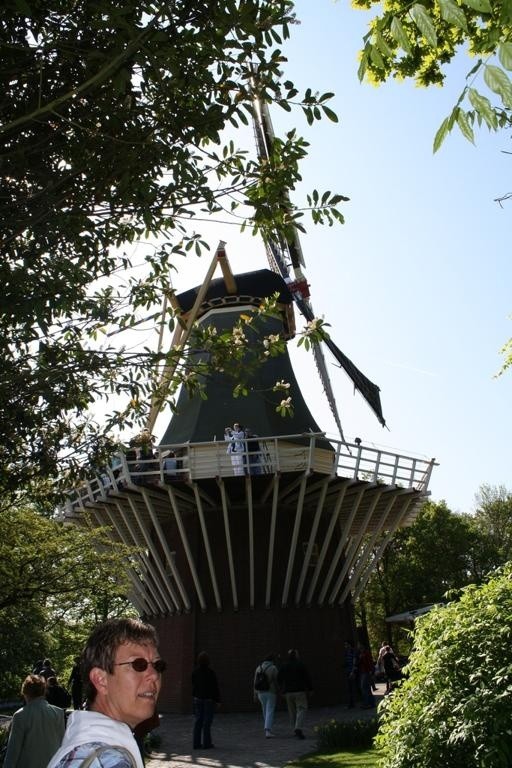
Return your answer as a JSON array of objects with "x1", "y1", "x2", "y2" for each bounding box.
[
  {"x1": 266, "y1": 731, "x2": 276, "y2": 737},
  {"x1": 294, "y1": 728, "x2": 304, "y2": 739},
  {"x1": 193, "y1": 744, "x2": 204, "y2": 749},
  {"x1": 202, "y1": 743, "x2": 214, "y2": 748}
]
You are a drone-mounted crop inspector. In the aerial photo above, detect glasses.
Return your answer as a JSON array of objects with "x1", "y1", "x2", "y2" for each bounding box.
[{"x1": 113, "y1": 658, "x2": 168, "y2": 673}]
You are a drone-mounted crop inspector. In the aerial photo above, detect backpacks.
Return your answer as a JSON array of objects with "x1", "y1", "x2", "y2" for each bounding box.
[{"x1": 255, "y1": 664, "x2": 273, "y2": 691}]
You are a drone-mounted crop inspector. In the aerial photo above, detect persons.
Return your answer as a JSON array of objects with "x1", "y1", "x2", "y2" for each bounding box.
[
  {"x1": 190, "y1": 650, "x2": 225, "y2": 750},
  {"x1": 223, "y1": 423, "x2": 248, "y2": 477},
  {"x1": 106, "y1": 442, "x2": 178, "y2": 490},
  {"x1": 46, "y1": 616, "x2": 164, "y2": 768},
  {"x1": 32, "y1": 659, "x2": 45, "y2": 675},
  {"x1": 253, "y1": 652, "x2": 284, "y2": 738},
  {"x1": 41, "y1": 659, "x2": 56, "y2": 677},
  {"x1": 44, "y1": 677, "x2": 69, "y2": 710},
  {"x1": 1, "y1": 675, "x2": 66, "y2": 768},
  {"x1": 340, "y1": 639, "x2": 400, "y2": 710},
  {"x1": 67, "y1": 656, "x2": 85, "y2": 711},
  {"x1": 241, "y1": 426, "x2": 263, "y2": 476},
  {"x1": 276, "y1": 648, "x2": 316, "y2": 740}
]
